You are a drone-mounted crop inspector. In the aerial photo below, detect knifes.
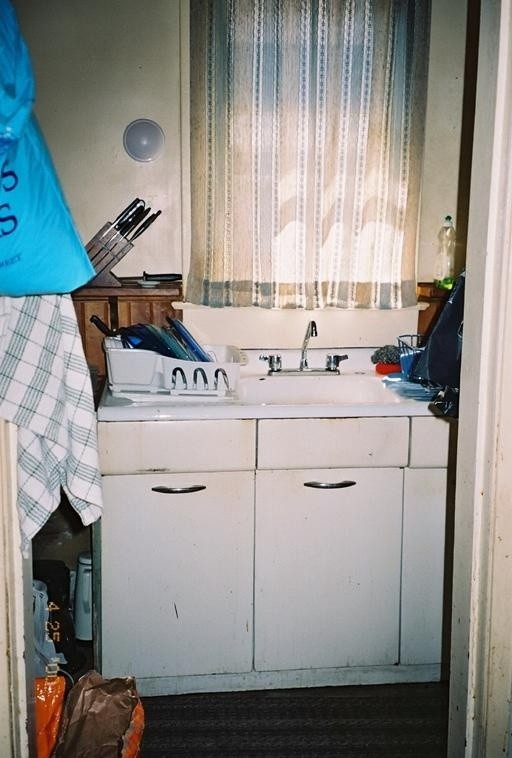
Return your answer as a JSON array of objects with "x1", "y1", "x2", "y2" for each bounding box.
[
  {"x1": 117, "y1": 271, "x2": 185, "y2": 284},
  {"x1": 89, "y1": 314, "x2": 117, "y2": 337},
  {"x1": 112, "y1": 197, "x2": 163, "y2": 243}
]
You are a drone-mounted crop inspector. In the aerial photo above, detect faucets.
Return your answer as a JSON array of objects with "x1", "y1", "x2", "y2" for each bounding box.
[{"x1": 301, "y1": 321, "x2": 317, "y2": 370}]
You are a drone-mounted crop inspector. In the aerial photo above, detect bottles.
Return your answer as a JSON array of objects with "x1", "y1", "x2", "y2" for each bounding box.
[{"x1": 72, "y1": 551, "x2": 93, "y2": 642}]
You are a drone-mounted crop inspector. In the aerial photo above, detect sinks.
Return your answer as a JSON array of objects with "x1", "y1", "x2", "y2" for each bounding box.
[{"x1": 236, "y1": 375, "x2": 401, "y2": 405}]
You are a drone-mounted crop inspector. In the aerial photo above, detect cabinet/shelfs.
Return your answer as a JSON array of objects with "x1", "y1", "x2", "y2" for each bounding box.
[{"x1": 97, "y1": 418, "x2": 448, "y2": 700}]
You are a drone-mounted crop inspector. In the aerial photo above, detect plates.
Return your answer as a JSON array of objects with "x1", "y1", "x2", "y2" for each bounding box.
[
  {"x1": 138, "y1": 280, "x2": 160, "y2": 287},
  {"x1": 120, "y1": 317, "x2": 215, "y2": 363}
]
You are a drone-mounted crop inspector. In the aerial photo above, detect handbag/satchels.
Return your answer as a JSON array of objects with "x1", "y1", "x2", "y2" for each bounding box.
[
  {"x1": 409, "y1": 269, "x2": 466, "y2": 385},
  {"x1": 0, "y1": 1, "x2": 97, "y2": 295},
  {"x1": 34, "y1": 663, "x2": 145, "y2": 758}
]
